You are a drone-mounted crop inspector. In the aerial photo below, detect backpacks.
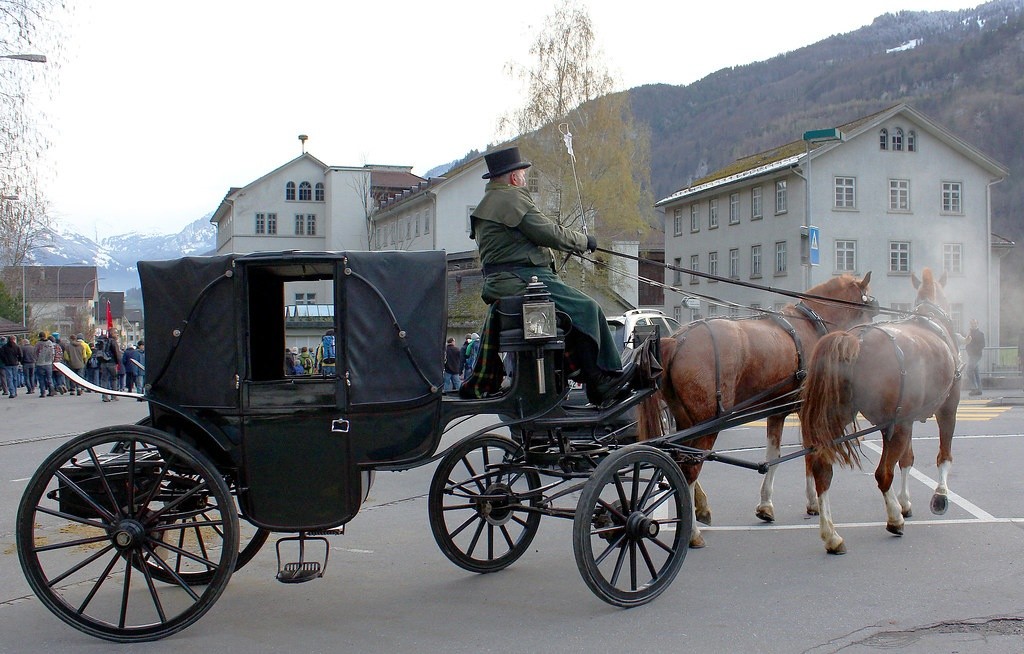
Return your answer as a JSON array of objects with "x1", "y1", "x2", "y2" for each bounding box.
[
  {"x1": 89, "y1": 354, "x2": 98, "y2": 368},
  {"x1": 301, "y1": 355, "x2": 312, "y2": 370},
  {"x1": 324, "y1": 336, "x2": 336, "y2": 364},
  {"x1": 97, "y1": 339, "x2": 114, "y2": 361}
]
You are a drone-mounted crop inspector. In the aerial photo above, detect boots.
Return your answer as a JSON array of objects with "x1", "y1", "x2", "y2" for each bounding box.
[{"x1": 593, "y1": 361, "x2": 638, "y2": 402}]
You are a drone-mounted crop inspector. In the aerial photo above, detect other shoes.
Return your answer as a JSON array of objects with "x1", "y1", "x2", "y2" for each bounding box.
[
  {"x1": 968, "y1": 389, "x2": 982, "y2": 396},
  {"x1": 102, "y1": 397, "x2": 120, "y2": 403},
  {"x1": 0, "y1": 383, "x2": 91, "y2": 398}
]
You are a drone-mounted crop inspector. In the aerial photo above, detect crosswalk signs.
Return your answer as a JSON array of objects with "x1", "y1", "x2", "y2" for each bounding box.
[{"x1": 808, "y1": 225, "x2": 820, "y2": 266}]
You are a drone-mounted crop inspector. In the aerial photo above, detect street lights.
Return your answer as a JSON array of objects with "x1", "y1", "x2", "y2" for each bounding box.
[
  {"x1": 57, "y1": 262, "x2": 84, "y2": 335},
  {"x1": 800, "y1": 127, "x2": 847, "y2": 297},
  {"x1": 82, "y1": 277, "x2": 107, "y2": 338},
  {"x1": 22, "y1": 244, "x2": 57, "y2": 328}
]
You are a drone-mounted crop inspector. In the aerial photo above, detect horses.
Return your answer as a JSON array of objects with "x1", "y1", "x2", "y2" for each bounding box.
[
  {"x1": 803, "y1": 267, "x2": 961, "y2": 555},
  {"x1": 639, "y1": 271, "x2": 874, "y2": 547}
]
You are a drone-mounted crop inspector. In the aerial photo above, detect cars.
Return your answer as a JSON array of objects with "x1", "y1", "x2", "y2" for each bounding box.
[{"x1": 565, "y1": 308, "x2": 684, "y2": 392}]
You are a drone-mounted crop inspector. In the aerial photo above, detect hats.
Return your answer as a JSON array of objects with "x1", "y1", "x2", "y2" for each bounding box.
[
  {"x1": 481, "y1": 145, "x2": 531, "y2": 179},
  {"x1": 138, "y1": 341, "x2": 145, "y2": 346},
  {"x1": 77, "y1": 333, "x2": 84, "y2": 339},
  {"x1": 292, "y1": 347, "x2": 298, "y2": 353},
  {"x1": 309, "y1": 348, "x2": 314, "y2": 352},
  {"x1": 465, "y1": 333, "x2": 472, "y2": 339},
  {"x1": 302, "y1": 347, "x2": 307, "y2": 351},
  {"x1": 39, "y1": 332, "x2": 45, "y2": 337},
  {"x1": 471, "y1": 333, "x2": 479, "y2": 339}
]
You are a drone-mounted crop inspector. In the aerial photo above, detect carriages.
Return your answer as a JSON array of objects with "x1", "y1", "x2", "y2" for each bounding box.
[{"x1": 15, "y1": 247, "x2": 963, "y2": 645}]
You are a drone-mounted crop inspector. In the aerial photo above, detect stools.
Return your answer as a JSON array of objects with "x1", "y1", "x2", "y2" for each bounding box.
[{"x1": 496, "y1": 296, "x2": 571, "y2": 348}]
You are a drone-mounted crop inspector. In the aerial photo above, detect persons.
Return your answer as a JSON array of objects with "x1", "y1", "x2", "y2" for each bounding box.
[
  {"x1": 285, "y1": 330, "x2": 335, "y2": 375},
  {"x1": 469, "y1": 146, "x2": 637, "y2": 407},
  {"x1": 966, "y1": 319, "x2": 983, "y2": 395},
  {"x1": 460, "y1": 333, "x2": 481, "y2": 381},
  {"x1": 0, "y1": 328, "x2": 146, "y2": 402},
  {"x1": 443, "y1": 337, "x2": 461, "y2": 391},
  {"x1": 1018, "y1": 328, "x2": 1024, "y2": 377}
]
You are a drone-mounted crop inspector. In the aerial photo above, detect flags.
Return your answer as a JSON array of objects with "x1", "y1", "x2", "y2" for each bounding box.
[{"x1": 107, "y1": 298, "x2": 113, "y2": 331}]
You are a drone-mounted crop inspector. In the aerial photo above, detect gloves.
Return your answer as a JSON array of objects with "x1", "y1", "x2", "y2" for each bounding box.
[
  {"x1": 459, "y1": 369, "x2": 463, "y2": 376},
  {"x1": 586, "y1": 235, "x2": 597, "y2": 253}
]
